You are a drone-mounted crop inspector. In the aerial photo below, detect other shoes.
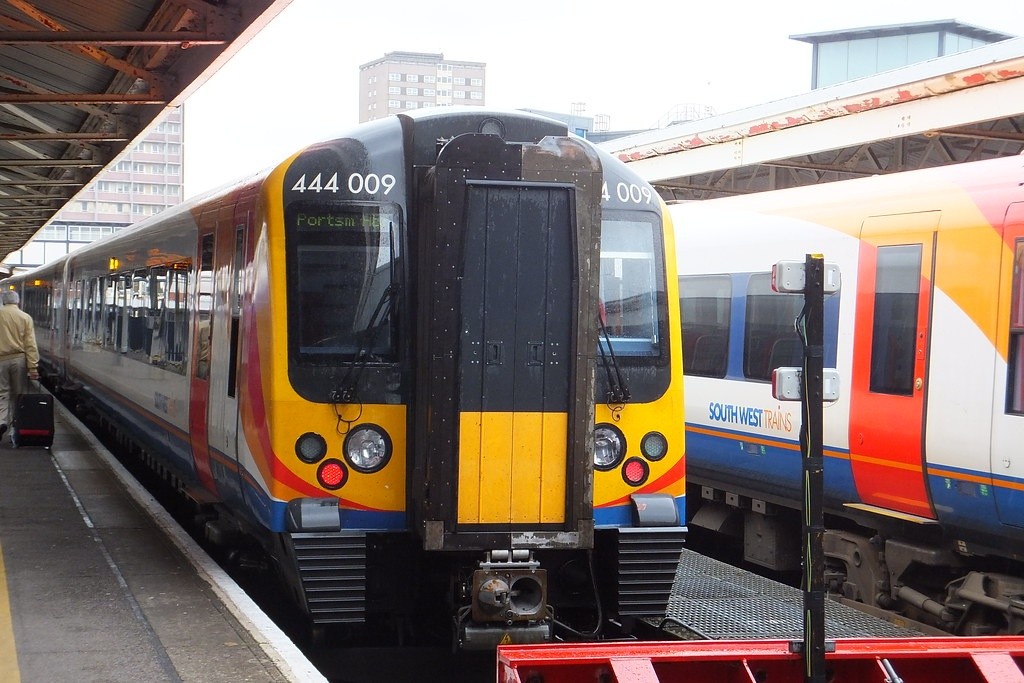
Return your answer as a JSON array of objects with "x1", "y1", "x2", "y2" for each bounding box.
[{"x1": 0, "y1": 424, "x2": 8, "y2": 442}]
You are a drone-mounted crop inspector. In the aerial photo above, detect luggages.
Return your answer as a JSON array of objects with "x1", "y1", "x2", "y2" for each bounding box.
[{"x1": 15, "y1": 376, "x2": 55, "y2": 449}]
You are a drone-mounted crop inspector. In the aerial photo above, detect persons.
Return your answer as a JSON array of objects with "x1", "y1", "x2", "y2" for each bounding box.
[
  {"x1": 195, "y1": 310, "x2": 210, "y2": 379},
  {"x1": 0, "y1": 290, "x2": 40, "y2": 441}
]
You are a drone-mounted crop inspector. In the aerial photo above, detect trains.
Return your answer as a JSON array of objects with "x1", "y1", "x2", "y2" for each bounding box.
[
  {"x1": 598, "y1": 34, "x2": 1024, "y2": 640},
  {"x1": 1, "y1": 106, "x2": 687, "y2": 683}
]
